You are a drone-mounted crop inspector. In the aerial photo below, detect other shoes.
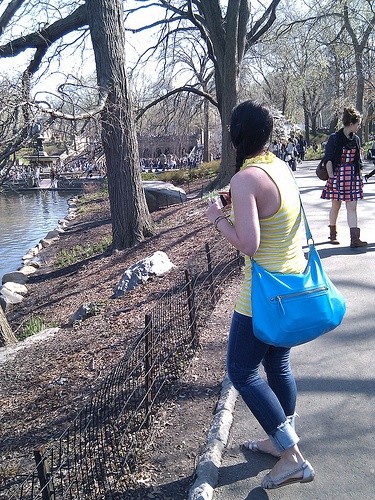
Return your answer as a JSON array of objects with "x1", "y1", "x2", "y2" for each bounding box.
[
  {"x1": 243, "y1": 440, "x2": 281, "y2": 458},
  {"x1": 364, "y1": 175, "x2": 367, "y2": 182},
  {"x1": 260, "y1": 460, "x2": 315, "y2": 489}
]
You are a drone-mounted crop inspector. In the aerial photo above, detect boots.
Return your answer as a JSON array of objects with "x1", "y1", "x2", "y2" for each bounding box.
[
  {"x1": 350, "y1": 227, "x2": 368, "y2": 248},
  {"x1": 328, "y1": 225, "x2": 339, "y2": 244}
]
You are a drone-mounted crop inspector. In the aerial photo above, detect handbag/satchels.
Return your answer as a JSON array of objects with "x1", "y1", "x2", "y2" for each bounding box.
[
  {"x1": 315, "y1": 156, "x2": 330, "y2": 180},
  {"x1": 251, "y1": 245, "x2": 346, "y2": 348}
]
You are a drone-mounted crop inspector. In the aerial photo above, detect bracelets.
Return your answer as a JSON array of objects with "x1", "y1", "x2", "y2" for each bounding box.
[{"x1": 214, "y1": 215, "x2": 232, "y2": 233}]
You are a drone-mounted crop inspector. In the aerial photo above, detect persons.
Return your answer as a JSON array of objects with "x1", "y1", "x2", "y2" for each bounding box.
[
  {"x1": 206, "y1": 99, "x2": 316, "y2": 489},
  {"x1": 364, "y1": 140, "x2": 375, "y2": 182},
  {"x1": 269, "y1": 135, "x2": 307, "y2": 171},
  {"x1": 320, "y1": 107, "x2": 368, "y2": 248},
  {"x1": 0, "y1": 152, "x2": 220, "y2": 188}
]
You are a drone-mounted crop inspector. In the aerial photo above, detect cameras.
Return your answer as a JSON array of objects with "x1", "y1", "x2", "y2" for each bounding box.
[{"x1": 214, "y1": 195, "x2": 230, "y2": 209}]
